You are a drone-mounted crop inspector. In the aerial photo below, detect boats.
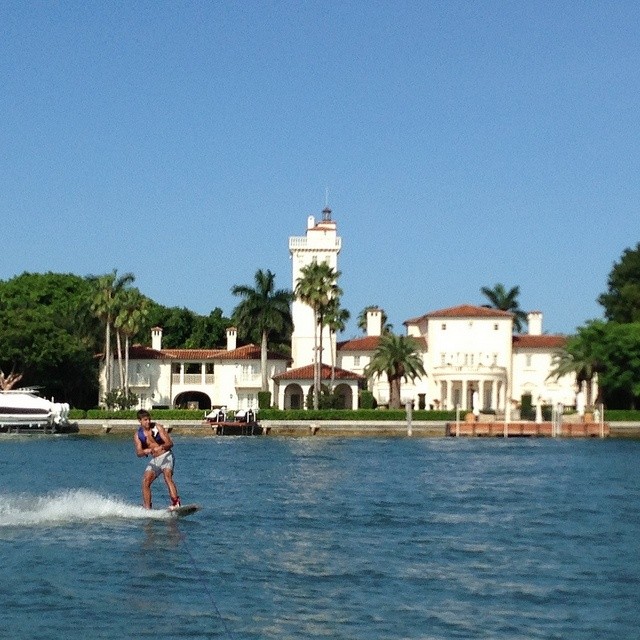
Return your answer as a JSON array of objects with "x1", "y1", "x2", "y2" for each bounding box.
[{"x1": 0, "y1": 390, "x2": 70, "y2": 434}]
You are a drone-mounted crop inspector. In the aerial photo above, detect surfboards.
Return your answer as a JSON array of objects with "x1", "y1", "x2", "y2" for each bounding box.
[{"x1": 167, "y1": 504, "x2": 203, "y2": 518}]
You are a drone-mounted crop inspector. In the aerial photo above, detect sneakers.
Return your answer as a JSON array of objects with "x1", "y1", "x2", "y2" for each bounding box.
[{"x1": 168, "y1": 501, "x2": 181, "y2": 510}]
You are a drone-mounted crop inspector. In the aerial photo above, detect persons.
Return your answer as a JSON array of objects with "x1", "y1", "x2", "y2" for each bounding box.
[
  {"x1": 133, "y1": 408, "x2": 181, "y2": 511},
  {"x1": 142, "y1": 519, "x2": 180, "y2": 551},
  {"x1": 0, "y1": 363, "x2": 24, "y2": 390}
]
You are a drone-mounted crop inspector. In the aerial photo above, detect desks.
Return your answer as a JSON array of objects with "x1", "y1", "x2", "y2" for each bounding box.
[{"x1": 207, "y1": 422, "x2": 256, "y2": 436}]
